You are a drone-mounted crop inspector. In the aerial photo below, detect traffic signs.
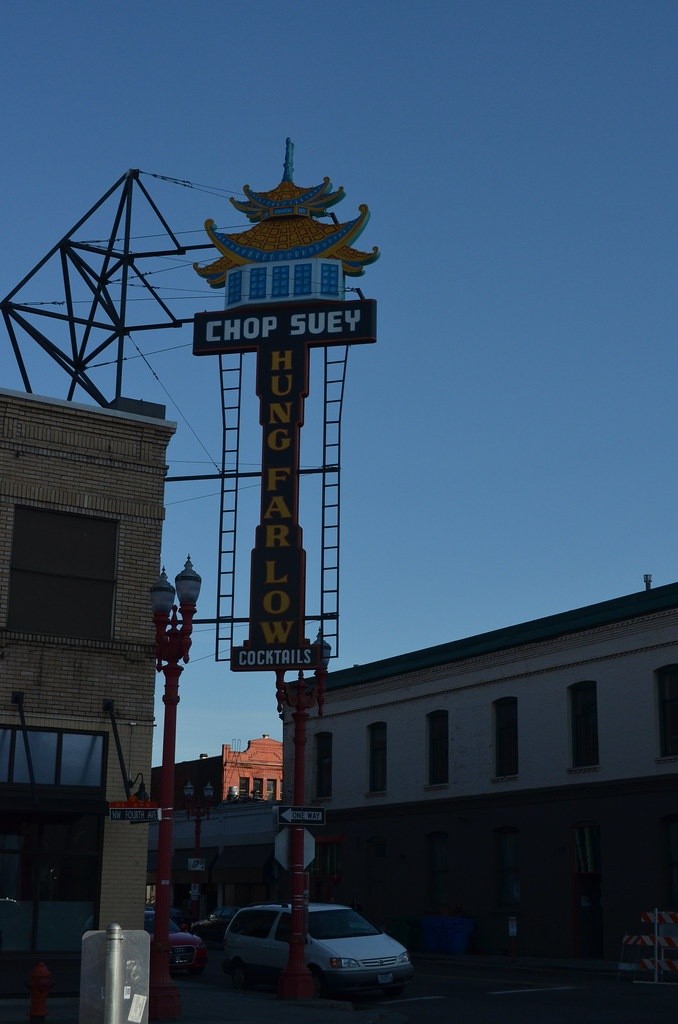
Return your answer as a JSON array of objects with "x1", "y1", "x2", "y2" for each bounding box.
[
  {"x1": 111, "y1": 807, "x2": 162, "y2": 822},
  {"x1": 273, "y1": 805, "x2": 326, "y2": 826}
]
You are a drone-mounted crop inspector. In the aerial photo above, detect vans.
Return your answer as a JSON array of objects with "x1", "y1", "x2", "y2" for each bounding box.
[{"x1": 223, "y1": 899, "x2": 414, "y2": 999}]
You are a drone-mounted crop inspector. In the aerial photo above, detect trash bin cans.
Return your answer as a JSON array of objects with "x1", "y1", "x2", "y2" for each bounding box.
[
  {"x1": 420, "y1": 916, "x2": 478, "y2": 955},
  {"x1": 391, "y1": 917, "x2": 424, "y2": 953},
  {"x1": 79, "y1": 930, "x2": 150, "y2": 1024}
]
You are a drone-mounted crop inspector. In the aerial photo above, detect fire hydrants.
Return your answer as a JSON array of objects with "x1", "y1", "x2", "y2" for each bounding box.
[{"x1": 24, "y1": 960, "x2": 52, "y2": 1024}]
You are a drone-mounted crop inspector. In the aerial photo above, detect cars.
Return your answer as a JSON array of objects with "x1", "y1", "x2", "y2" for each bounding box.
[
  {"x1": 145, "y1": 910, "x2": 208, "y2": 977},
  {"x1": 146, "y1": 905, "x2": 189, "y2": 932},
  {"x1": 191, "y1": 905, "x2": 242, "y2": 944}
]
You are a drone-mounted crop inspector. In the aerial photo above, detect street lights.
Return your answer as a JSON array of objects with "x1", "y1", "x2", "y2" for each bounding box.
[
  {"x1": 274, "y1": 625, "x2": 332, "y2": 1001},
  {"x1": 149, "y1": 556, "x2": 201, "y2": 1024},
  {"x1": 182, "y1": 779, "x2": 215, "y2": 924}
]
